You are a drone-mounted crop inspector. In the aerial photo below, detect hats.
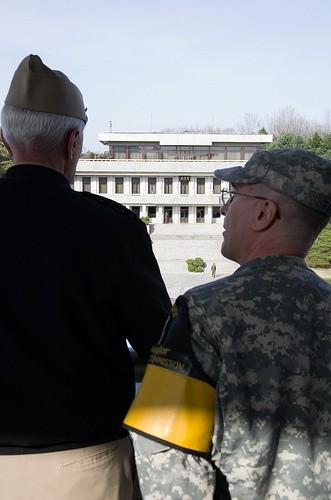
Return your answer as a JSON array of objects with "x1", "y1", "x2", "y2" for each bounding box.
[
  {"x1": 214, "y1": 148, "x2": 330, "y2": 216},
  {"x1": 4, "y1": 55, "x2": 89, "y2": 125}
]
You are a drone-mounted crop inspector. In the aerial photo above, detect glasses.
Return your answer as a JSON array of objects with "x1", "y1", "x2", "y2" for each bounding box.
[{"x1": 221, "y1": 188, "x2": 282, "y2": 220}]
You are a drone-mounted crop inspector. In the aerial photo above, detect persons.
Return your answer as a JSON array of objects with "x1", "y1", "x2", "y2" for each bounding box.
[
  {"x1": 1, "y1": 55, "x2": 174, "y2": 499},
  {"x1": 129, "y1": 144, "x2": 331, "y2": 499}
]
